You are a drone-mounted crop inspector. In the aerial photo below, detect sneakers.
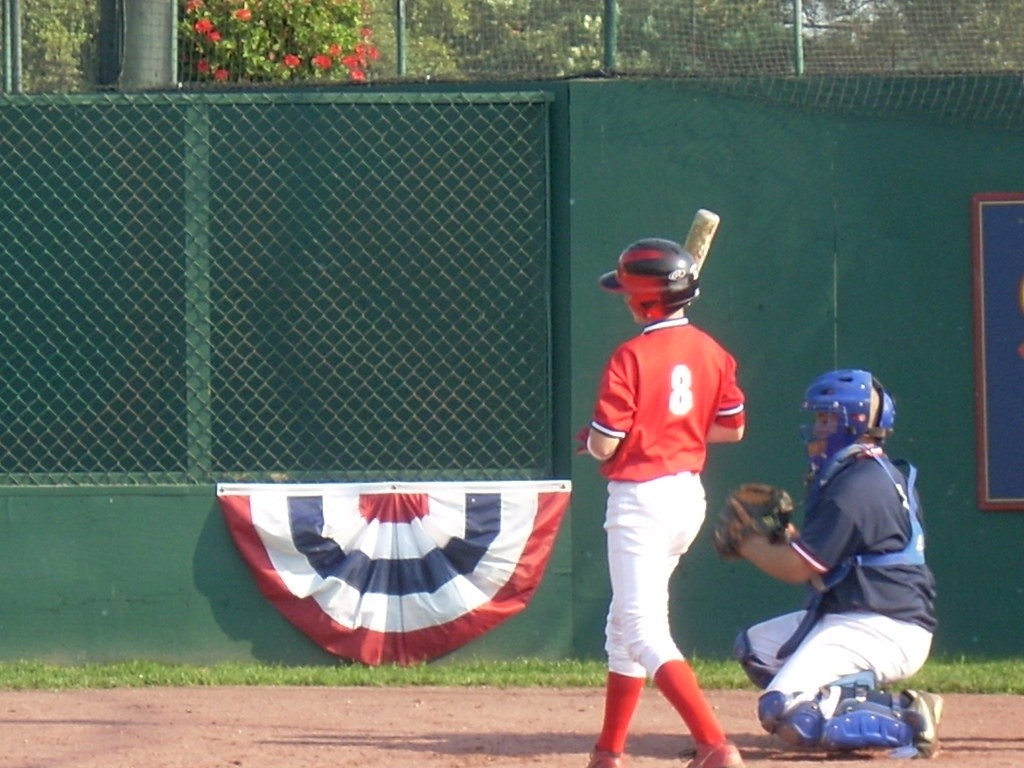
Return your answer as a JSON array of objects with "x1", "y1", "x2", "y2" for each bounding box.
[
  {"x1": 900, "y1": 688, "x2": 944, "y2": 760},
  {"x1": 679, "y1": 742, "x2": 744, "y2": 768},
  {"x1": 587, "y1": 747, "x2": 621, "y2": 768}
]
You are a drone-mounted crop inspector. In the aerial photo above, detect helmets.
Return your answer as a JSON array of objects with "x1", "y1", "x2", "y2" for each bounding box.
[
  {"x1": 597, "y1": 238, "x2": 699, "y2": 306},
  {"x1": 799, "y1": 369, "x2": 894, "y2": 470}
]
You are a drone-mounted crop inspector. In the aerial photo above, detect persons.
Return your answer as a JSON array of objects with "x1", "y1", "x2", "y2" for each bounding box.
[
  {"x1": 578, "y1": 239, "x2": 748, "y2": 768},
  {"x1": 715, "y1": 369, "x2": 944, "y2": 760}
]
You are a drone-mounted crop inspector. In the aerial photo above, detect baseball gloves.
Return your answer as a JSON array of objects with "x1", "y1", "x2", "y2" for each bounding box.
[{"x1": 711, "y1": 482, "x2": 794, "y2": 560}]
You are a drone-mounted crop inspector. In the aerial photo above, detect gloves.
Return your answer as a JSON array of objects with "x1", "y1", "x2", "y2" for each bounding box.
[{"x1": 576, "y1": 427, "x2": 590, "y2": 452}]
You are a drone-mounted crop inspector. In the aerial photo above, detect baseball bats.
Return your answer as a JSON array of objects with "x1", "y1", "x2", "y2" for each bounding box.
[{"x1": 683, "y1": 207, "x2": 721, "y2": 271}]
